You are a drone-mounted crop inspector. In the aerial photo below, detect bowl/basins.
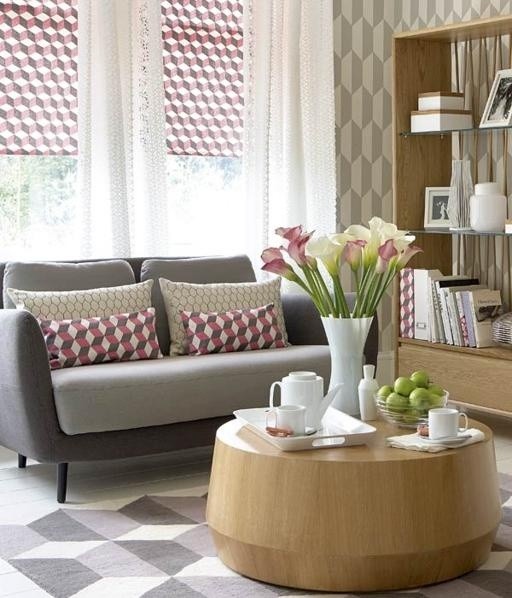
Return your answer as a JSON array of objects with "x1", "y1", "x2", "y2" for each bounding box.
[{"x1": 371, "y1": 390, "x2": 450, "y2": 428}]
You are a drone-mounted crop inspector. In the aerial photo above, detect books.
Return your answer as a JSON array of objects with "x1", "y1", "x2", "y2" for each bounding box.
[{"x1": 427, "y1": 276, "x2": 504, "y2": 348}]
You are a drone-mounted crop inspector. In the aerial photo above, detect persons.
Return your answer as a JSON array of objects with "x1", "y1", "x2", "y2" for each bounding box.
[{"x1": 440, "y1": 201, "x2": 447, "y2": 220}]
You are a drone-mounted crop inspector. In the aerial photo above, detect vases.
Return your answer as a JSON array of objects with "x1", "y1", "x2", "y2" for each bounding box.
[
  {"x1": 392, "y1": 13, "x2": 512, "y2": 419},
  {"x1": 320, "y1": 314, "x2": 373, "y2": 417}
]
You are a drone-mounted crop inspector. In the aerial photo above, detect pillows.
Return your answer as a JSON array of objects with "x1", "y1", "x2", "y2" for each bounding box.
[
  {"x1": 180, "y1": 301, "x2": 289, "y2": 356},
  {"x1": 36, "y1": 308, "x2": 163, "y2": 370},
  {"x1": 158, "y1": 276, "x2": 289, "y2": 357},
  {"x1": 6, "y1": 279, "x2": 154, "y2": 322}
]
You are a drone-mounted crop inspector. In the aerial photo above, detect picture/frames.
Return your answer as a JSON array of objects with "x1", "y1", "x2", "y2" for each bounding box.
[
  {"x1": 479, "y1": 68, "x2": 512, "y2": 128},
  {"x1": 423, "y1": 187, "x2": 459, "y2": 228}
]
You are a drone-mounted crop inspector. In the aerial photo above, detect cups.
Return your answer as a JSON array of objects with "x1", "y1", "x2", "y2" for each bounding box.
[
  {"x1": 265, "y1": 404, "x2": 307, "y2": 435},
  {"x1": 427, "y1": 407, "x2": 468, "y2": 441}
]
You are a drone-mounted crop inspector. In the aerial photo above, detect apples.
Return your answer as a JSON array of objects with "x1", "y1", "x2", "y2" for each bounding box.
[
  {"x1": 386, "y1": 392, "x2": 408, "y2": 413},
  {"x1": 403, "y1": 409, "x2": 420, "y2": 423},
  {"x1": 409, "y1": 371, "x2": 436, "y2": 390},
  {"x1": 377, "y1": 385, "x2": 393, "y2": 401},
  {"x1": 409, "y1": 388, "x2": 432, "y2": 411},
  {"x1": 394, "y1": 376, "x2": 416, "y2": 397},
  {"x1": 425, "y1": 394, "x2": 444, "y2": 412},
  {"x1": 427, "y1": 384, "x2": 446, "y2": 396}
]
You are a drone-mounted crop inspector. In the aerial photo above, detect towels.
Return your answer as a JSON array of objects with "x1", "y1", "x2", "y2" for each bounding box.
[{"x1": 386, "y1": 429, "x2": 483, "y2": 453}]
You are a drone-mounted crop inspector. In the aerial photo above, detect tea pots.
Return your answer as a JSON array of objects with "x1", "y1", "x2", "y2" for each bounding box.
[{"x1": 269, "y1": 370, "x2": 344, "y2": 431}]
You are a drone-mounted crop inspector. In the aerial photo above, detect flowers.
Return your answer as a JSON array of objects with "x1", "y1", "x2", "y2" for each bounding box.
[{"x1": 260, "y1": 217, "x2": 424, "y2": 319}]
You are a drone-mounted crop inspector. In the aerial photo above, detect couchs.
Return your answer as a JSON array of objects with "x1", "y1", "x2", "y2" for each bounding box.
[{"x1": 0, "y1": 253, "x2": 277, "y2": 504}]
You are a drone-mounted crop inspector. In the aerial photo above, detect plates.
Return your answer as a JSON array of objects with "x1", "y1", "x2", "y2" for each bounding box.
[{"x1": 232, "y1": 404, "x2": 377, "y2": 452}]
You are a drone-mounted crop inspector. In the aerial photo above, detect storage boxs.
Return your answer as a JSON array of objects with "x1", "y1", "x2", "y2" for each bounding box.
[
  {"x1": 418, "y1": 91, "x2": 464, "y2": 110},
  {"x1": 410, "y1": 110, "x2": 473, "y2": 133}
]
